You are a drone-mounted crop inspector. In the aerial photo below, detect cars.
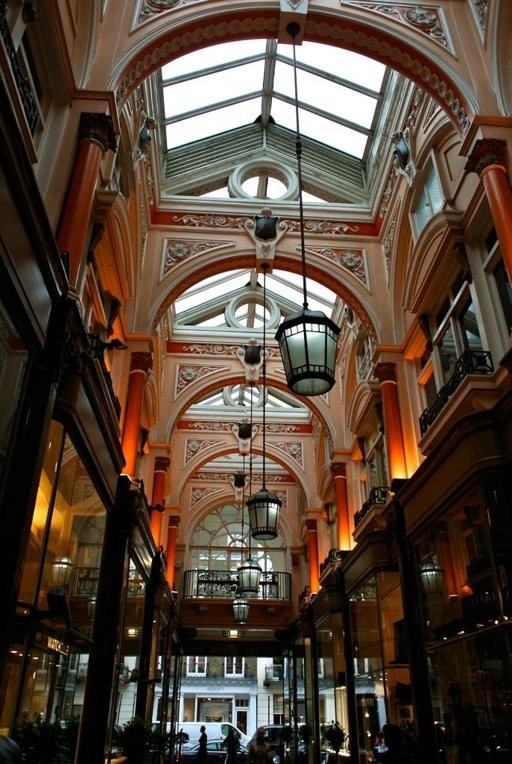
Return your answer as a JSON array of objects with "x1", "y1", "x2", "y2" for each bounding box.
[{"x1": 177, "y1": 739, "x2": 249, "y2": 764}]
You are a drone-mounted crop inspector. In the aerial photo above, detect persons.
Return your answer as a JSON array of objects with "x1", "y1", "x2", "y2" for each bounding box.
[
  {"x1": 221, "y1": 731, "x2": 240, "y2": 764},
  {"x1": 381, "y1": 725, "x2": 408, "y2": 764},
  {"x1": 199, "y1": 726, "x2": 207, "y2": 764},
  {"x1": 248, "y1": 728, "x2": 270, "y2": 764}
]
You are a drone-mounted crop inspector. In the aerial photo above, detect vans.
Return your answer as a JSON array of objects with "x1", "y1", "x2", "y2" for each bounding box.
[{"x1": 151, "y1": 721, "x2": 252, "y2": 748}]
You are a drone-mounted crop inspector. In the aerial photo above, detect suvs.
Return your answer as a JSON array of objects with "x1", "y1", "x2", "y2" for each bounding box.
[{"x1": 247, "y1": 725, "x2": 282, "y2": 764}]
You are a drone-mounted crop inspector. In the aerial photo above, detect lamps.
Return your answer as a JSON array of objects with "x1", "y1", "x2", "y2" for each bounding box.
[
  {"x1": 245, "y1": 263, "x2": 284, "y2": 541},
  {"x1": 237, "y1": 378, "x2": 262, "y2": 598},
  {"x1": 228, "y1": 629, "x2": 242, "y2": 640},
  {"x1": 86, "y1": 596, "x2": 97, "y2": 622},
  {"x1": 231, "y1": 454, "x2": 251, "y2": 624},
  {"x1": 274, "y1": 21, "x2": 342, "y2": 399},
  {"x1": 49, "y1": 553, "x2": 76, "y2": 595},
  {"x1": 420, "y1": 552, "x2": 445, "y2": 596},
  {"x1": 127, "y1": 627, "x2": 137, "y2": 638}
]
